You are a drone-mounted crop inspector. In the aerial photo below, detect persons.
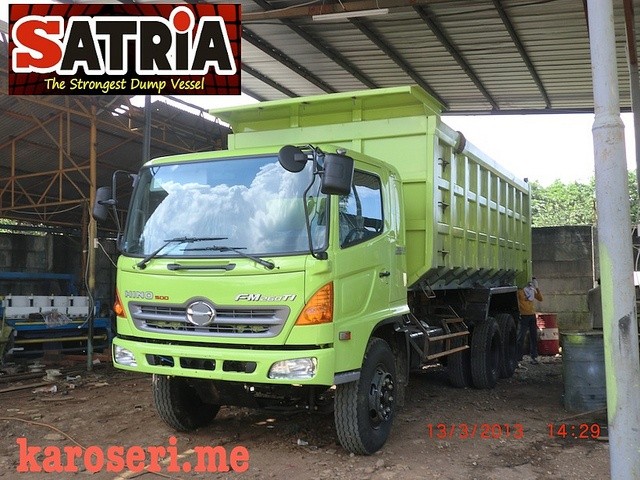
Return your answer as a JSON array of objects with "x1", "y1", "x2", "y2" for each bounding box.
[
  {"x1": 586, "y1": 278, "x2": 603, "y2": 330},
  {"x1": 518, "y1": 276, "x2": 543, "y2": 365}
]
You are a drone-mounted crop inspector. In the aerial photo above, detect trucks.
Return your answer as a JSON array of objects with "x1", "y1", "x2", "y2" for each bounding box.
[{"x1": 91, "y1": 83, "x2": 533, "y2": 456}]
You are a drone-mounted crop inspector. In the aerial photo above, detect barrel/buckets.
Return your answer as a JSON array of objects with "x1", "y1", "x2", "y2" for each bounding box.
[
  {"x1": 561, "y1": 329, "x2": 607, "y2": 412},
  {"x1": 536, "y1": 312, "x2": 559, "y2": 358}
]
